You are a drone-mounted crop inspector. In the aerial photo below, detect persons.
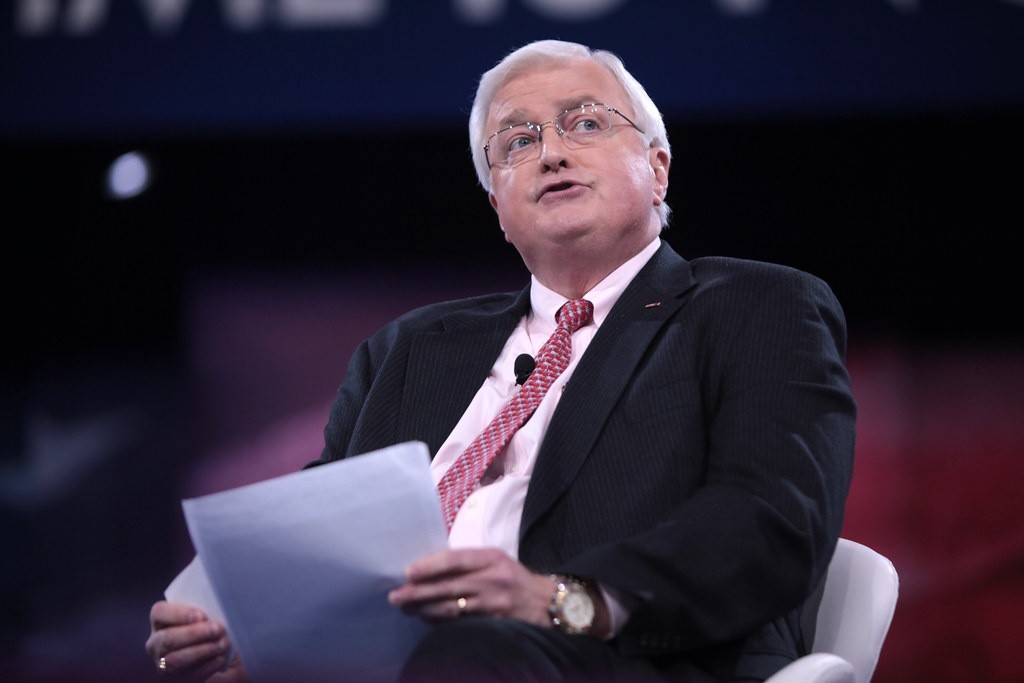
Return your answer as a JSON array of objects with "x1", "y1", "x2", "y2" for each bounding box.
[{"x1": 144, "y1": 38, "x2": 854, "y2": 683}]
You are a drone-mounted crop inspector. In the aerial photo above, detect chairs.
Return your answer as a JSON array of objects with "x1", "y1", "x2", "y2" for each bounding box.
[{"x1": 759, "y1": 539, "x2": 898, "y2": 683}]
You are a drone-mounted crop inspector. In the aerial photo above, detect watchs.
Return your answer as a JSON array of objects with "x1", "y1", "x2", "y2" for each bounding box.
[{"x1": 547, "y1": 573, "x2": 596, "y2": 636}]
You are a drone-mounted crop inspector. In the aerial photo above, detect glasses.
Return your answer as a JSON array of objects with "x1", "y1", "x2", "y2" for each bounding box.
[{"x1": 483, "y1": 103, "x2": 655, "y2": 171}]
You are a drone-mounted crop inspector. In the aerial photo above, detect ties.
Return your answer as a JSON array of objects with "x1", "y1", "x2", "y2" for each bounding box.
[{"x1": 436, "y1": 299, "x2": 594, "y2": 538}]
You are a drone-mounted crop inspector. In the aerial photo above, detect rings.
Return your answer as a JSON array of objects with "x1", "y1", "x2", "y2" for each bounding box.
[
  {"x1": 159, "y1": 657, "x2": 168, "y2": 672},
  {"x1": 458, "y1": 598, "x2": 466, "y2": 616}
]
